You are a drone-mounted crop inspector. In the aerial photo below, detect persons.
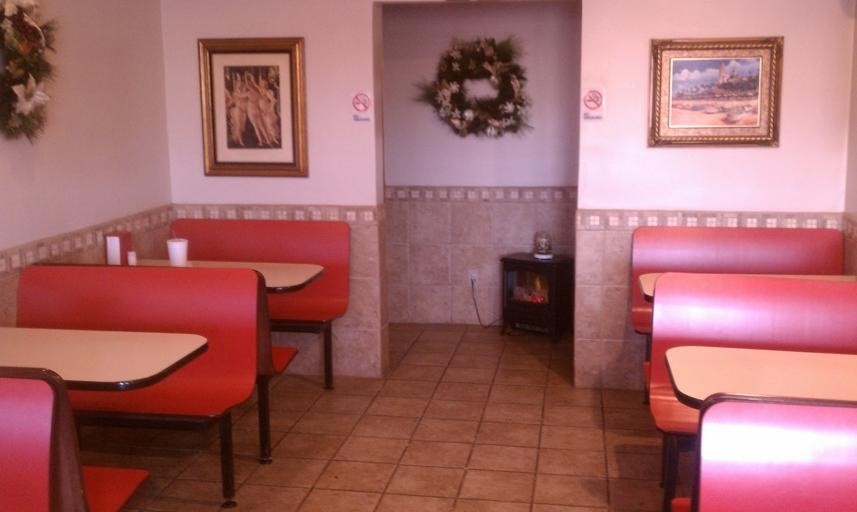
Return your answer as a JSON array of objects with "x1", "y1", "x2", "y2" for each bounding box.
[{"x1": 225, "y1": 70, "x2": 281, "y2": 147}]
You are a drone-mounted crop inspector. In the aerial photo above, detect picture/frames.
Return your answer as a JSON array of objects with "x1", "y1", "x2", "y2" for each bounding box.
[
  {"x1": 197, "y1": 38, "x2": 308, "y2": 177},
  {"x1": 651, "y1": 37, "x2": 784, "y2": 147}
]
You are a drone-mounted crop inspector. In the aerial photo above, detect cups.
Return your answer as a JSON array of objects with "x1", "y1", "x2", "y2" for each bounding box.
[{"x1": 166, "y1": 238, "x2": 188, "y2": 268}]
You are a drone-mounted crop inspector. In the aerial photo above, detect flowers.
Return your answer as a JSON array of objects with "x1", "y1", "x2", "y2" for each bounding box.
[
  {"x1": 410, "y1": 36, "x2": 536, "y2": 139},
  {"x1": 0, "y1": 0, "x2": 57, "y2": 147}
]
